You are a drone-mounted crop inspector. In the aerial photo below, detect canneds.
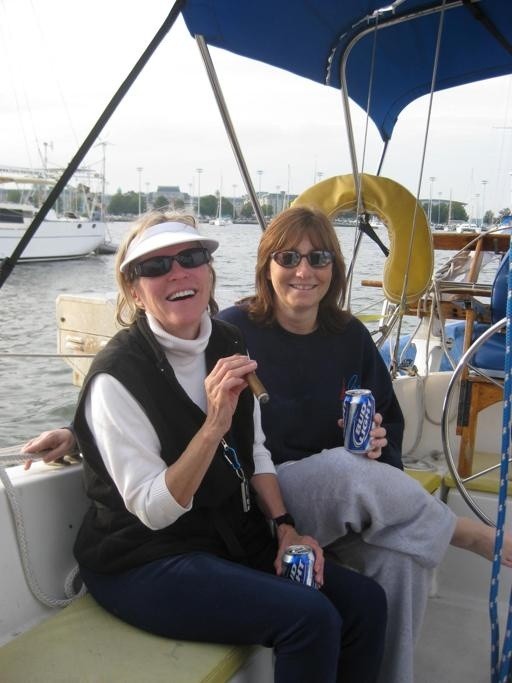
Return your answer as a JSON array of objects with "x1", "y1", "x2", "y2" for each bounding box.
[
  {"x1": 281, "y1": 544, "x2": 318, "y2": 590},
  {"x1": 342, "y1": 388, "x2": 376, "y2": 454}
]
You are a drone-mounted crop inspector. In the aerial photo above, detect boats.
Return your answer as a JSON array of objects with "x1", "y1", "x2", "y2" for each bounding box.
[
  {"x1": 1, "y1": 0, "x2": 510, "y2": 678},
  {"x1": 0, "y1": 140, "x2": 119, "y2": 263}
]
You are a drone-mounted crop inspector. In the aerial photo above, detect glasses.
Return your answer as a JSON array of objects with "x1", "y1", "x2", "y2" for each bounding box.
[
  {"x1": 270, "y1": 250, "x2": 335, "y2": 268},
  {"x1": 129, "y1": 248, "x2": 213, "y2": 281}
]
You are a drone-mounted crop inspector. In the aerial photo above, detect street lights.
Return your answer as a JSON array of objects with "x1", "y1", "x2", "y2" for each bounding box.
[
  {"x1": 232, "y1": 171, "x2": 323, "y2": 220},
  {"x1": 137, "y1": 167, "x2": 143, "y2": 216},
  {"x1": 426, "y1": 175, "x2": 488, "y2": 227},
  {"x1": 196, "y1": 168, "x2": 202, "y2": 214}
]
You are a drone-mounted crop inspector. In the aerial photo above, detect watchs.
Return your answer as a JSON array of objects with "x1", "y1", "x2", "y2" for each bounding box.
[{"x1": 272, "y1": 513, "x2": 296, "y2": 531}]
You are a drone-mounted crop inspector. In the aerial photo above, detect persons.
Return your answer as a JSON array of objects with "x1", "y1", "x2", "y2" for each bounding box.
[
  {"x1": 16, "y1": 207, "x2": 512, "y2": 683},
  {"x1": 72, "y1": 212, "x2": 387, "y2": 681}
]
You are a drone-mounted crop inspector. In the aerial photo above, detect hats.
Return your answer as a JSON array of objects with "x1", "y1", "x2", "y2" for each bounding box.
[{"x1": 120, "y1": 222, "x2": 219, "y2": 274}]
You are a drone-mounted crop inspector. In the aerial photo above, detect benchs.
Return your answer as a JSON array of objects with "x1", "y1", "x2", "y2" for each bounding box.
[{"x1": 0, "y1": 429, "x2": 443, "y2": 675}]
